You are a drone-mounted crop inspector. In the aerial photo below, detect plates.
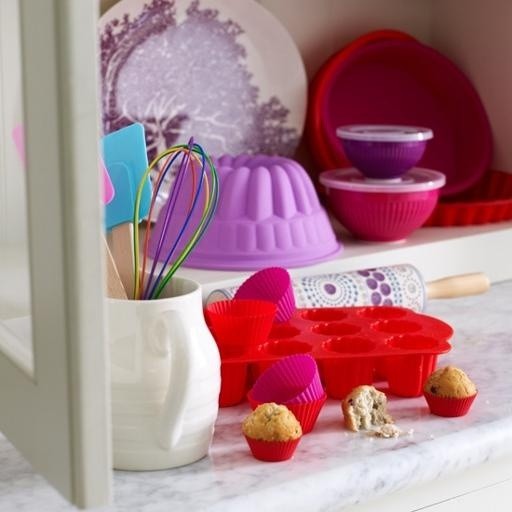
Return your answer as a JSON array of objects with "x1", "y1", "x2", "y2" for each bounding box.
[{"x1": 98, "y1": 2, "x2": 310, "y2": 184}]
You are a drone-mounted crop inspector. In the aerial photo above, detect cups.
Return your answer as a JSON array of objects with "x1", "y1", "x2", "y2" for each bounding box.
[
  {"x1": 204, "y1": 298, "x2": 279, "y2": 352},
  {"x1": 106, "y1": 275, "x2": 222, "y2": 471},
  {"x1": 252, "y1": 352, "x2": 326, "y2": 402},
  {"x1": 246, "y1": 390, "x2": 329, "y2": 435},
  {"x1": 230, "y1": 267, "x2": 302, "y2": 316},
  {"x1": 244, "y1": 427, "x2": 302, "y2": 464},
  {"x1": 417, "y1": 389, "x2": 477, "y2": 421}
]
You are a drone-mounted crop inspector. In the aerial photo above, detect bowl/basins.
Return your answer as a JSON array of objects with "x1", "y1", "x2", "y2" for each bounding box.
[
  {"x1": 323, "y1": 39, "x2": 493, "y2": 200},
  {"x1": 316, "y1": 166, "x2": 448, "y2": 247},
  {"x1": 144, "y1": 155, "x2": 347, "y2": 273},
  {"x1": 335, "y1": 124, "x2": 435, "y2": 184},
  {"x1": 306, "y1": 27, "x2": 428, "y2": 170}
]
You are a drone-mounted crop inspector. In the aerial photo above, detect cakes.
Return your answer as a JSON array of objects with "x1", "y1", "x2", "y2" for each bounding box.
[
  {"x1": 243, "y1": 402, "x2": 304, "y2": 462},
  {"x1": 343, "y1": 383, "x2": 402, "y2": 440},
  {"x1": 424, "y1": 365, "x2": 480, "y2": 417}
]
